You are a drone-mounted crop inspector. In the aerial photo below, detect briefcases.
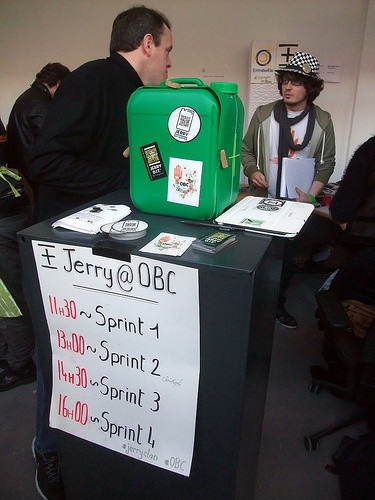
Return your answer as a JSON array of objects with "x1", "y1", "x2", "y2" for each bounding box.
[{"x1": 128, "y1": 77, "x2": 245, "y2": 218}]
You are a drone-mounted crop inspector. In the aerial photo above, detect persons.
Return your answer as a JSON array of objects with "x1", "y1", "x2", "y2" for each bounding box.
[
  {"x1": 0, "y1": 121, "x2": 38, "y2": 393},
  {"x1": 316, "y1": 135, "x2": 375, "y2": 307},
  {"x1": 25, "y1": 3, "x2": 173, "y2": 222},
  {"x1": 235, "y1": 51, "x2": 336, "y2": 331},
  {"x1": 7, "y1": 62, "x2": 72, "y2": 211}
]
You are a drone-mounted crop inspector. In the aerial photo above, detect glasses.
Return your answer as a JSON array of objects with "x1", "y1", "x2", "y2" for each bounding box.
[{"x1": 279, "y1": 77, "x2": 305, "y2": 86}]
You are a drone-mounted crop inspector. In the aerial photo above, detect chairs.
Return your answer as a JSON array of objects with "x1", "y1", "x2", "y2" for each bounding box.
[{"x1": 303, "y1": 290, "x2": 375, "y2": 452}]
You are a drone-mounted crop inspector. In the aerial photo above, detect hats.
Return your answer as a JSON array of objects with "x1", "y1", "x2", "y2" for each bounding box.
[{"x1": 274, "y1": 50, "x2": 324, "y2": 81}]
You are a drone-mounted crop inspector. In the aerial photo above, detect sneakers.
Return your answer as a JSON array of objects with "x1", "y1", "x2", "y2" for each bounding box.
[
  {"x1": 31, "y1": 435, "x2": 67, "y2": 500},
  {"x1": 275, "y1": 296, "x2": 298, "y2": 330}
]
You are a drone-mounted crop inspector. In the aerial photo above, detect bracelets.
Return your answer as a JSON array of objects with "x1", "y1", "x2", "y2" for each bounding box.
[{"x1": 306, "y1": 190, "x2": 318, "y2": 207}]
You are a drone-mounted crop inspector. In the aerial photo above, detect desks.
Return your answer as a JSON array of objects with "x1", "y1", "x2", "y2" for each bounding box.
[{"x1": 15, "y1": 190, "x2": 285, "y2": 500}]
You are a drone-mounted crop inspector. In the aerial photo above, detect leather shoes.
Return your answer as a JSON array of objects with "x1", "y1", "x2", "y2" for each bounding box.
[{"x1": 0, "y1": 360, "x2": 37, "y2": 392}]
